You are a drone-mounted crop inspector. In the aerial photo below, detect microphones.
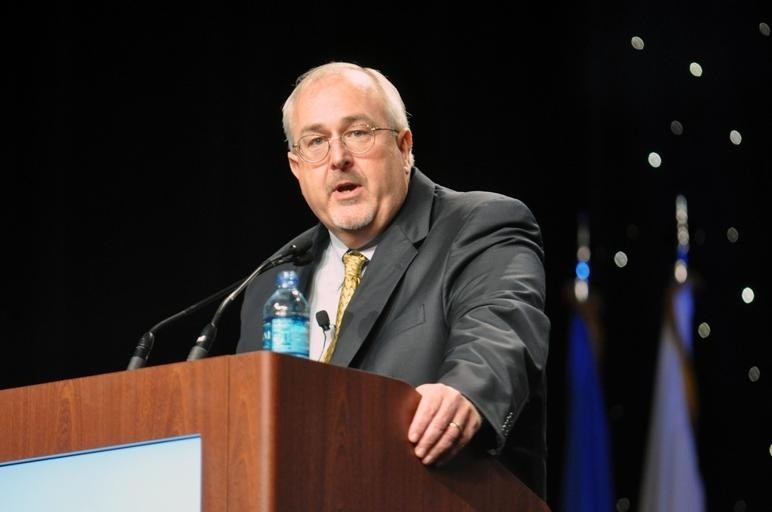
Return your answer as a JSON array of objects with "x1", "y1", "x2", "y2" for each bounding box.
[
  {"x1": 125, "y1": 251, "x2": 316, "y2": 371},
  {"x1": 187, "y1": 235, "x2": 313, "y2": 360},
  {"x1": 316, "y1": 310, "x2": 330, "y2": 361}
]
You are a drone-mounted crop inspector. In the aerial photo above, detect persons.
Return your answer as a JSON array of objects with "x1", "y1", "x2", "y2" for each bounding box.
[{"x1": 234, "y1": 62, "x2": 553, "y2": 466}]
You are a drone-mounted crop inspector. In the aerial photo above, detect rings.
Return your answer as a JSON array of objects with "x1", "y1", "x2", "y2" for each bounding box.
[{"x1": 450, "y1": 421, "x2": 464, "y2": 434}]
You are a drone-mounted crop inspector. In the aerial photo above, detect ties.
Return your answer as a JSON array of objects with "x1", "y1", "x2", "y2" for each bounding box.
[{"x1": 324, "y1": 251, "x2": 368, "y2": 365}]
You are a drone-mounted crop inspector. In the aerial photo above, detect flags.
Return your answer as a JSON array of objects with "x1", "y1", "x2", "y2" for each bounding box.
[
  {"x1": 564, "y1": 278, "x2": 621, "y2": 510},
  {"x1": 636, "y1": 268, "x2": 702, "y2": 510}
]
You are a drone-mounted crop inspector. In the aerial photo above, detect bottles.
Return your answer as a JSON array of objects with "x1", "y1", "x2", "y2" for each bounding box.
[{"x1": 261, "y1": 269, "x2": 311, "y2": 362}]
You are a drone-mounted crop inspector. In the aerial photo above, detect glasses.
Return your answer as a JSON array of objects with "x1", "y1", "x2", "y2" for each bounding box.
[{"x1": 290, "y1": 124, "x2": 398, "y2": 166}]
